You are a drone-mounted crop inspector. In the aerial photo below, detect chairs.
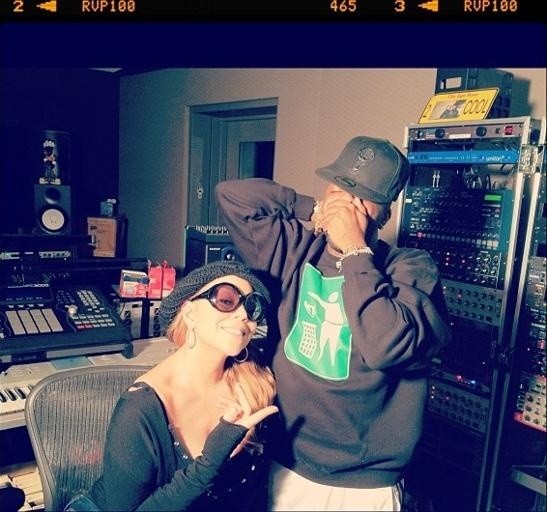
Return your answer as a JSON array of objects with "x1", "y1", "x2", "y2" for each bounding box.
[{"x1": 25, "y1": 365, "x2": 152, "y2": 512}]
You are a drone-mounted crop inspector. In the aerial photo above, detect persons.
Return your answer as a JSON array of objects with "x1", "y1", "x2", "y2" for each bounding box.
[
  {"x1": 63, "y1": 260, "x2": 288, "y2": 507},
  {"x1": 215, "y1": 135, "x2": 451, "y2": 507}
]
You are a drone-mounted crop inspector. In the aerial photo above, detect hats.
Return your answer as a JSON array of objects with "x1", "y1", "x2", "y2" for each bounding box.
[
  {"x1": 157, "y1": 260, "x2": 272, "y2": 331},
  {"x1": 315, "y1": 135, "x2": 410, "y2": 203}
]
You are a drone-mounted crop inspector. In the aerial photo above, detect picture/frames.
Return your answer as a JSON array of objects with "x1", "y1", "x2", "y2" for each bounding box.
[{"x1": 418, "y1": 87, "x2": 500, "y2": 124}]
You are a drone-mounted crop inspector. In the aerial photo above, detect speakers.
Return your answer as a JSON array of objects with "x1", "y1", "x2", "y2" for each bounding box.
[
  {"x1": 33, "y1": 183, "x2": 72, "y2": 239},
  {"x1": 184, "y1": 224, "x2": 245, "y2": 278}
]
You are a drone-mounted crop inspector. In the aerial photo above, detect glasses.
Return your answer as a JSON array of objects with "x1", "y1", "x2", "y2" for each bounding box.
[{"x1": 190, "y1": 281, "x2": 269, "y2": 322}]
